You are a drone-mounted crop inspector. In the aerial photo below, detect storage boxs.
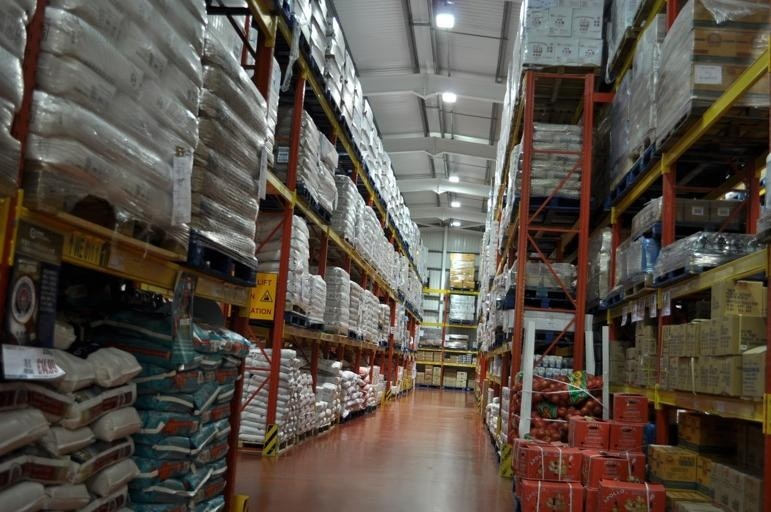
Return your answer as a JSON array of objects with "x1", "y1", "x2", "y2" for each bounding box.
[
  {"x1": 449, "y1": 253, "x2": 476, "y2": 321},
  {"x1": 500, "y1": 1, "x2": 771, "y2": 512},
  {"x1": 414, "y1": 350, "x2": 477, "y2": 390}
]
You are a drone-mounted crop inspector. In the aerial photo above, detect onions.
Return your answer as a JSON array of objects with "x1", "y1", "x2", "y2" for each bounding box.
[{"x1": 509, "y1": 376, "x2": 602, "y2": 443}]
